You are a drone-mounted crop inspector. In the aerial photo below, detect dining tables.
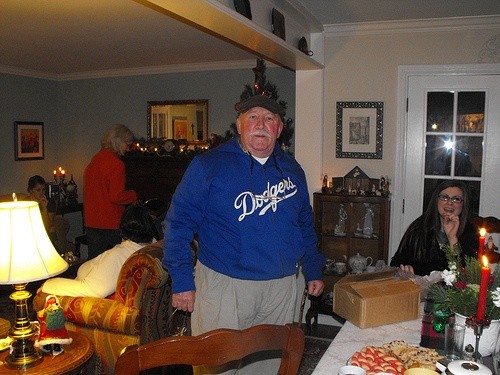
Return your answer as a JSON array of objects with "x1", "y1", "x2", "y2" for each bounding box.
[{"x1": 310, "y1": 302, "x2": 425, "y2": 375}]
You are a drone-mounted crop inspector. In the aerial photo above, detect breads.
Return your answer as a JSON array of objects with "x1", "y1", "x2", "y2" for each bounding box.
[{"x1": 389, "y1": 340, "x2": 441, "y2": 375}]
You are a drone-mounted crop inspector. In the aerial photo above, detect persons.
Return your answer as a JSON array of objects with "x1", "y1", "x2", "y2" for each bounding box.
[
  {"x1": 389, "y1": 180, "x2": 480, "y2": 275},
  {"x1": 162, "y1": 82, "x2": 325, "y2": 375},
  {"x1": 25, "y1": 124, "x2": 157, "y2": 297}
]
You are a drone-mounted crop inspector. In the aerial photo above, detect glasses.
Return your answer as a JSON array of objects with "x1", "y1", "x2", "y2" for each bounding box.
[{"x1": 438, "y1": 193, "x2": 464, "y2": 204}]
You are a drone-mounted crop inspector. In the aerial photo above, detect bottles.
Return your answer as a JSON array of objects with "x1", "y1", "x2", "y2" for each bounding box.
[
  {"x1": 493, "y1": 330, "x2": 500, "y2": 375},
  {"x1": 375, "y1": 260, "x2": 386, "y2": 272}
]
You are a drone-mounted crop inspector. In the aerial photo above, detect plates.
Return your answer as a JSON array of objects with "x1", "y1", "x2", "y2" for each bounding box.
[
  {"x1": 299, "y1": 36, "x2": 308, "y2": 55},
  {"x1": 233, "y1": 0, "x2": 251, "y2": 20},
  {"x1": 0, "y1": 339, "x2": 13, "y2": 352}
]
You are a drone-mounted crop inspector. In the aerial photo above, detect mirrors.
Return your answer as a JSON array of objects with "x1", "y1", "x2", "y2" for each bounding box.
[{"x1": 147, "y1": 99, "x2": 209, "y2": 144}]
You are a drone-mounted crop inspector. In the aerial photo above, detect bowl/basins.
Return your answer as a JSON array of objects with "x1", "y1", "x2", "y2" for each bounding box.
[{"x1": 404, "y1": 368, "x2": 440, "y2": 375}]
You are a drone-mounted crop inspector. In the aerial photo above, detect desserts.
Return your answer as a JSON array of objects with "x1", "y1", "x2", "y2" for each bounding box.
[{"x1": 352, "y1": 345, "x2": 407, "y2": 375}]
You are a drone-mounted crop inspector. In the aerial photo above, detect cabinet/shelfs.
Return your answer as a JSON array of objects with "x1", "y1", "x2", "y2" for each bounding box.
[{"x1": 315, "y1": 192, "x2": 389, "y2": 269}]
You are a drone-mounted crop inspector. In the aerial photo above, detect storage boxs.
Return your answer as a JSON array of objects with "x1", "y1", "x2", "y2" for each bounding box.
[{"x1": 332, "y1": 268, "x2": 420, "y2": 329}]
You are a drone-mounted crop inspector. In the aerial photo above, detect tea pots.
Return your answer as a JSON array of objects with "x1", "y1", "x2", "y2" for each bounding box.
[{"x1": 344, "y1": 251, "x2": 373, "y2": 274}]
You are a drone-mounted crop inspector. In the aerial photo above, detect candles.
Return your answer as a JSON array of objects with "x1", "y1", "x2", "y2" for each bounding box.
[
  {"x1": 476, "y1": 256, "x2": 490, "y2": 324},
  {"x1": 53, "y1": 167, "x2": 66, "y2": 180},
  {"x1": 479, "y1": 227, "x2": 486, "y2": 260}
]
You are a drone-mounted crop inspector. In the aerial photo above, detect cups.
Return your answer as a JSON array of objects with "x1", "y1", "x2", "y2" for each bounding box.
[
  {"x1": 366, "y1": 266, "x2": 375, "y2": 273},
  {"x1": 338, "y1": 366, "x2": 366, "y2": 375},
  {"x1": 444, "y1": 324, "x2": 466, "y2": 362},
  {"x1": 335, "y1": 263, "x2": 346, "y2": 275}
]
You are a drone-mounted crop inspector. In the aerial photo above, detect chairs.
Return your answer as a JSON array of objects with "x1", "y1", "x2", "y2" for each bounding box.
[{"x1": 115, "y1": 324, "x2": 306, "y2": 375}]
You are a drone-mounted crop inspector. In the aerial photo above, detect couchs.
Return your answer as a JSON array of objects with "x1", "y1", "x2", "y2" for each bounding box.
[{"x1": 32, "y1": 238, "x2": 200, "y2": 375}]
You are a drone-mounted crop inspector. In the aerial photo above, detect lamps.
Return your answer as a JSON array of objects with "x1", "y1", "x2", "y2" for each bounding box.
[{"x1": 0, "y1": 193, "x2": 69, "y2": 370}]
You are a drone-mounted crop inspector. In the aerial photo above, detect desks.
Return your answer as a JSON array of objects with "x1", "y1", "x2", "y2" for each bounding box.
[{"x1": 0, "y1": 330, "x2": 94, "y2": 375}]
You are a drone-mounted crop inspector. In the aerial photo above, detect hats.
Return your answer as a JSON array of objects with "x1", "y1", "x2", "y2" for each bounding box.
[{"x1": 234, "y1": 94, "x2": 286, "y2": 118}]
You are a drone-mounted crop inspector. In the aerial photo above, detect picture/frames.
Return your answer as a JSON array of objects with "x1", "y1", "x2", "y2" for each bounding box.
[
  {"x1": 15, "y1": 121, "x2": 45, "y2": 161},
  {"x1": 335, "y1": 101, "x2": 384, "y2": 159}
]
